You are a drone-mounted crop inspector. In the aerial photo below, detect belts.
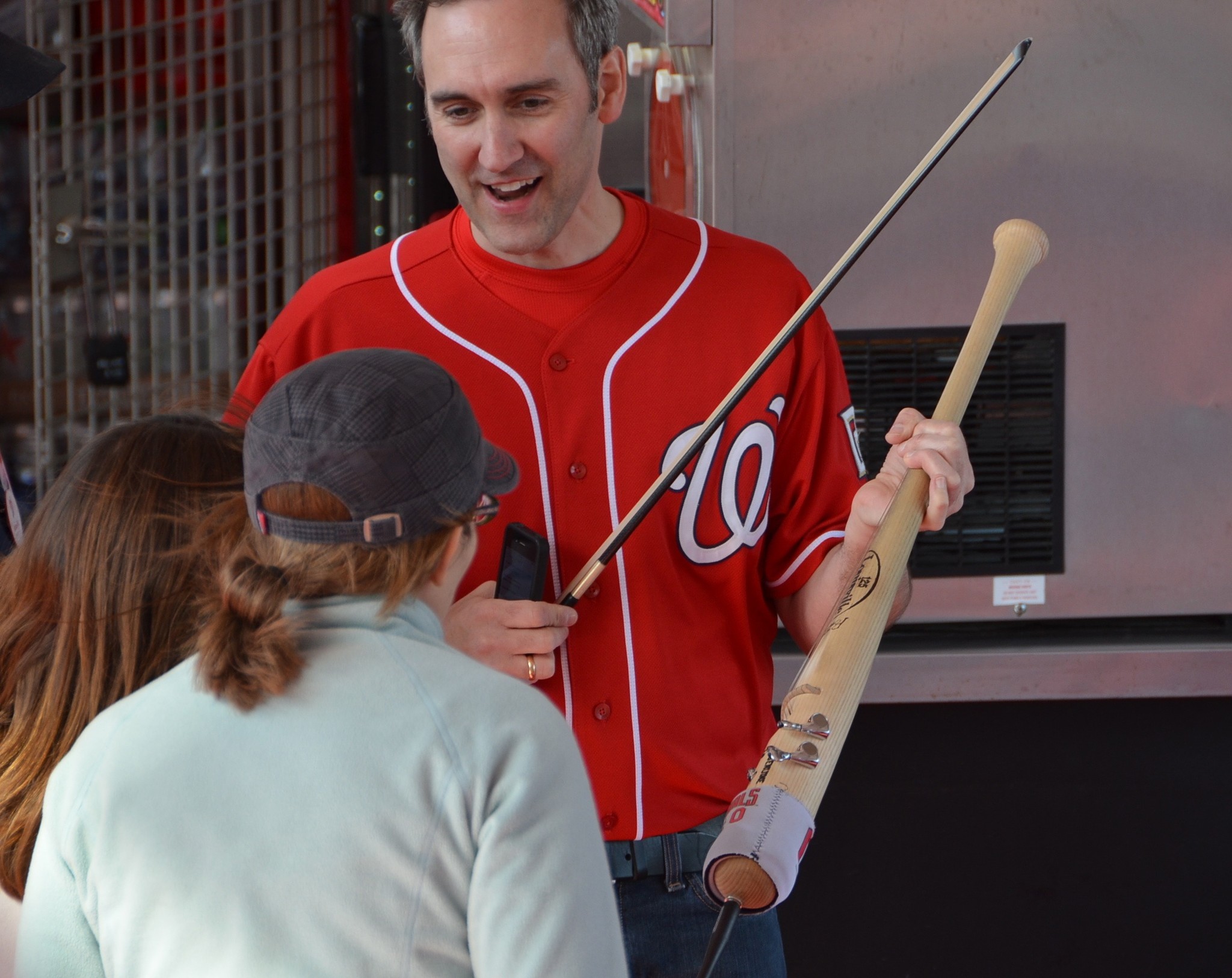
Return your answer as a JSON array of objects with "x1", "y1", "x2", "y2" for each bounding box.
[{"x1": 601, "y1": 832, "x2": 717, "y2": 880}]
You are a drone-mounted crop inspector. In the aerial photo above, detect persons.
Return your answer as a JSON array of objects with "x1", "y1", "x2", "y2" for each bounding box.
[
  {"x1": 0, "y1": 408, "x2": 296, "y2": 978},
  {"x1": 15, "y1": 343, "x2": 632, "y2": 978},
  {"x1": 214, "y1": 0, "x2": 976, "y2": 978}
]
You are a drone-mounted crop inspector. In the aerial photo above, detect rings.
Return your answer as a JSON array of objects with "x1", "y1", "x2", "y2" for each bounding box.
[{"x1": 525, "y1": 654, "x2": 537, "y2": 684}]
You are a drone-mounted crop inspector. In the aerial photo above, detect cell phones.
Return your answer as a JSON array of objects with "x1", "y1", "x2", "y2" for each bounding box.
[{"x1": 493, "y1": 522, "x2": 550, "y2": 603}]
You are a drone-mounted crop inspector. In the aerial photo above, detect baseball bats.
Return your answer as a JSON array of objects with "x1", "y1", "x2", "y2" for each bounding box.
[{"x1": 708, "y1": 217, "x2": 1050, "y2": 910}]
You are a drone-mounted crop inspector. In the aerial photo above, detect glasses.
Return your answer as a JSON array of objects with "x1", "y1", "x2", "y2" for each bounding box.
[{"x1": 469, "y1": 492, "x2": 499, "y2": 525}]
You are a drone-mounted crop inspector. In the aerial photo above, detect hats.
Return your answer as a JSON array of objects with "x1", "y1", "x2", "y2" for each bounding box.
[{"x1": 244, "y1": 346, "x2": 519, "y2": 546}]
